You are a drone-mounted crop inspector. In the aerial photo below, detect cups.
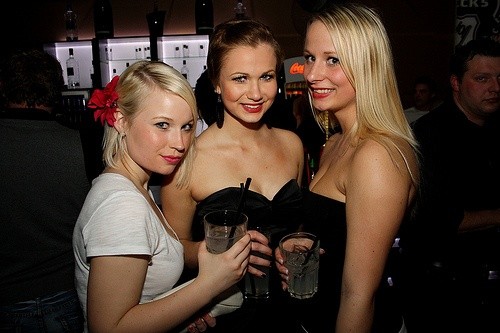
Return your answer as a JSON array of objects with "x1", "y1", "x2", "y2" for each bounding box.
[
  {"x1": 204, "y1": 209, "x2": 248, "y2": 255},
  {"x1": 237, "y1": 231, "x2": 271, "y2": 309},
  {"x1": 279, "y1": 232, "x2": 320, "y2": 299}
]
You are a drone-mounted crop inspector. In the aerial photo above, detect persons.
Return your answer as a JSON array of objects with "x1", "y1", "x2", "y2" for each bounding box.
[
  {"x1": 0, "y1": 47, "x2": 103, "y2": 333},
  {"x1": 160, "y1": 3, "x2": 500, "y2": 333},
  {"x1": 74, "y1": 60, "x2": 252, "y2": 333}
]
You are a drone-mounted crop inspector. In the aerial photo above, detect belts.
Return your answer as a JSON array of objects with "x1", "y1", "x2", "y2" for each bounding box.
[
  {"x1": 484, "y1": 270, "x2": 497, "y2": 285},
  {"x1": 8, "y1": 289, "x2": 75, "y2": 312}
]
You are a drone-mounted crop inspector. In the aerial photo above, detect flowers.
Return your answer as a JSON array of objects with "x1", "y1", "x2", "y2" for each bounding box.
[{"x1": 88, "y1": 74, "x2": 120, "y2": 128}]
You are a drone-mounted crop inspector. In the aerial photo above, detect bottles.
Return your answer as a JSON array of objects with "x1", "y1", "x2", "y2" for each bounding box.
[
  {"x1": 286, "y1": 82, "x2": 308, "y2": 100},
  {"x1": 310, "y1": 158, "x2": 315, "y2": 180},
  {"x1": 63, "y1": 0, "x2": 215, "y2": 90}
]
